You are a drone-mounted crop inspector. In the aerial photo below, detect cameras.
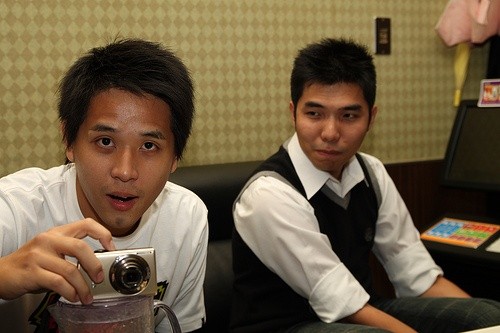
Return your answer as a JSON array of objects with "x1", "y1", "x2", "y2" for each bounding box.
[{"x1": 77, "y1": 247, "x2": 157, "y2": 301}]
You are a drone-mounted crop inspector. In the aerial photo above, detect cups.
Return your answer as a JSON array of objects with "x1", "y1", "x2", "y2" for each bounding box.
[{"x1": 46, "y1": 300, "x2": 182, "y2": 333}]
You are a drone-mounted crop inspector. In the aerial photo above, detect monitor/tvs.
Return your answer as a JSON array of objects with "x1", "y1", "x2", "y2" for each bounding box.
[{"x1": 437, "y1": 99, "x2": 500, "y2": 191}]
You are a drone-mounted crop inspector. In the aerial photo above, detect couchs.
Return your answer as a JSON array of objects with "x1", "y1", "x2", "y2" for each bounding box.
[{"x1": 166, "y1": 161, "x2": 261, "y2": 333}]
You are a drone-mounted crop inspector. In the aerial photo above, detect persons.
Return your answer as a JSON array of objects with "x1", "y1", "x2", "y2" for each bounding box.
[
  {"x1": 0, "y1": 34, "x2": 209, "y2": 333},
  {"x1": 232, "y1": 37, "x2": 500, "y2": 333}
]
